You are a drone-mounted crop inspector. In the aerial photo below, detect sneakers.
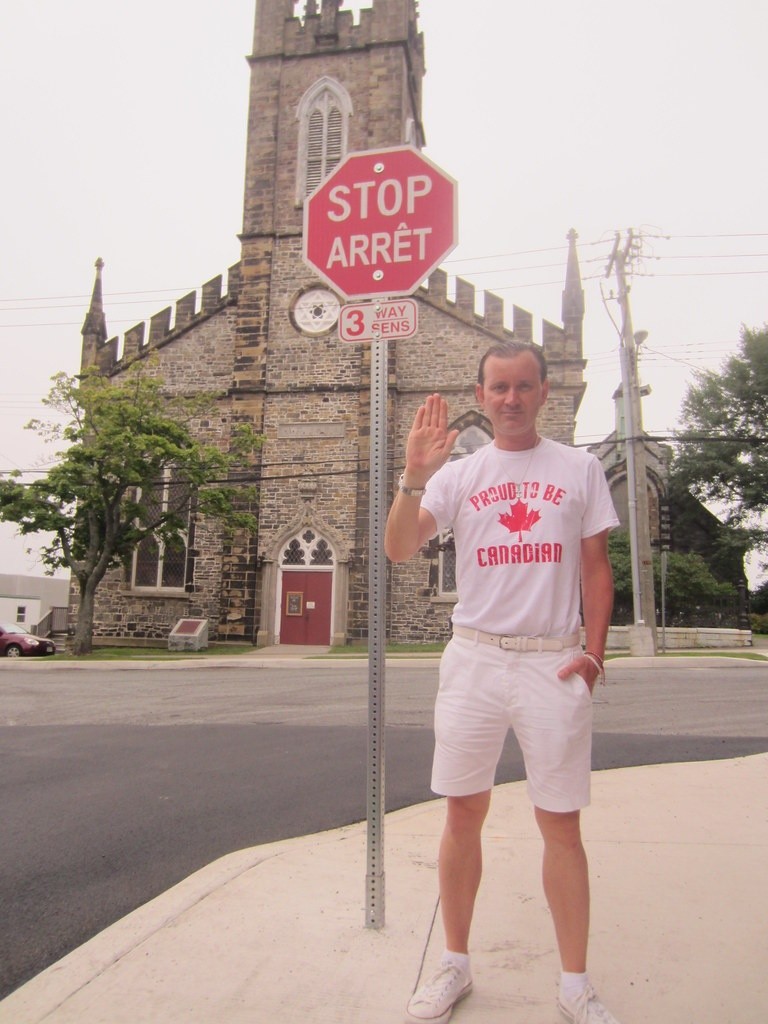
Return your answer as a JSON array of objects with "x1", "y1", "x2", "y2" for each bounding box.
[
  {"x1": 405, "y1": 959, "x2": 472, "y2": 1024},
  {"x1": 556, "y1": 983, "x2": 619, "y2": 1024}
]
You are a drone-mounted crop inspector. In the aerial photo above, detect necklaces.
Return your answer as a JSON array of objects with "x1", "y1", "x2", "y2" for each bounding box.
[{"x1": 495, "y1": 435, "x2": 539, "y2": 499}]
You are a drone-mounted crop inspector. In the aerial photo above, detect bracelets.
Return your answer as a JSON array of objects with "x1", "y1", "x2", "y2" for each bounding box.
[
  {"x1": 584, "y1": 654, "x2": 602, "y2": 673},
  {"x1": 584, "y1": 651, "x2": 607, "y2": 686},
  {"x1": 398, "y1": 474, "x2": 426, "y2": 496}
]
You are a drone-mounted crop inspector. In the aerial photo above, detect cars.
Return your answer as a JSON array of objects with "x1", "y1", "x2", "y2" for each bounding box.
[{"x1": 0, "y1": 620, "x2": 58, "y2": 658}]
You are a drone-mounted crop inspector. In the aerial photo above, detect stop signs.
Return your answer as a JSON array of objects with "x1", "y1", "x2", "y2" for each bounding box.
[{"x1": 304, "y1": 142, "x2": 460, "y2": 299}]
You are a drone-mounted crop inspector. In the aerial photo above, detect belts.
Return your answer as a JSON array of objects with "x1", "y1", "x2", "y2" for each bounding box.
[{"x1": 452, "y1": 624, "x2": 578, "y2": 652}]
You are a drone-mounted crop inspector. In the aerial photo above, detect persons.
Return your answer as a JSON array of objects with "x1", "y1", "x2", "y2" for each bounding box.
[{"x1": 383, "y1": 340, "x2": 621, "y2": 1024}]
